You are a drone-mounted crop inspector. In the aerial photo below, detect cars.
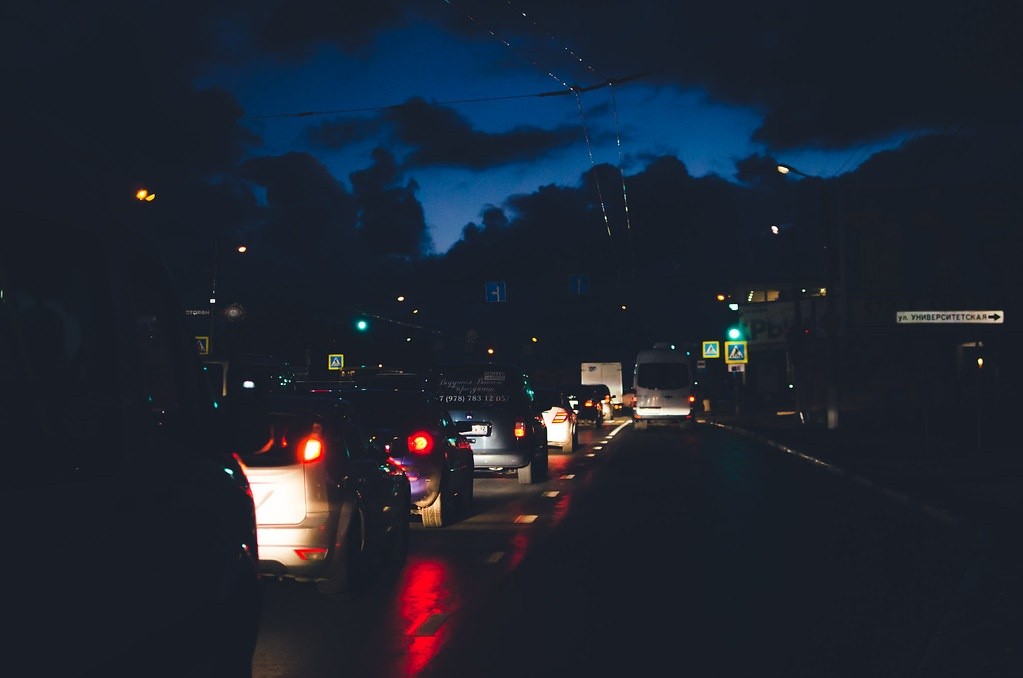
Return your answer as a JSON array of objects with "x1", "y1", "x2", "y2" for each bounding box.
[
  {"x1": 523, "y1": 383, "x2": 579, "y2": 452},
  {"x1": 563, "y1": 385, "x2": 604, "y2": 429},
  {"x1": 584, "y1": 384, "x2": 616, "y2": 423},
  {"x1": 316, "y1": 387, "x2": 475, "y2": 531},
  {"x1": 215, "y1": 393, "x2": 413, "y2": 607}
]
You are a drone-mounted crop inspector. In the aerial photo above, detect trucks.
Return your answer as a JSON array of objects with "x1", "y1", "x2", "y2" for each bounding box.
[{"x1": 581, "y1": 361, "x2": 624, "y2": 412}]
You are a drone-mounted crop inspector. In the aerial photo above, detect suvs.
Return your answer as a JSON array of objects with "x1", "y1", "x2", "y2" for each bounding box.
[
  {"x1": 0, "y1": 274, "x2": 263, "y2": 678},
  {"x1": 416, "y1": 364, "x2": 549, "y2": 485}
]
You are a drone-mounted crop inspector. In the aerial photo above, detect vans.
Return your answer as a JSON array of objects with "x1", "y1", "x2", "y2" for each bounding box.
[{"x1": 632, "y1": 351, "x2": 697, "y2": 430}]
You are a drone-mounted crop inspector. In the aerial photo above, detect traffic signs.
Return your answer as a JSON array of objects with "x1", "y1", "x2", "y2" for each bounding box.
[{"x1": 896, "y1": 308, "x2": 1005, "y2": 325}]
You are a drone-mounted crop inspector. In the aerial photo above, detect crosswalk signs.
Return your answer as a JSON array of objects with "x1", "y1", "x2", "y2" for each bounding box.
[
  {"x1": 725, "y1": 340, "x2": 748, "y2": 363},
  {"x1": 329, "y1": 355, "x2": 344, "y2": 370}
]
